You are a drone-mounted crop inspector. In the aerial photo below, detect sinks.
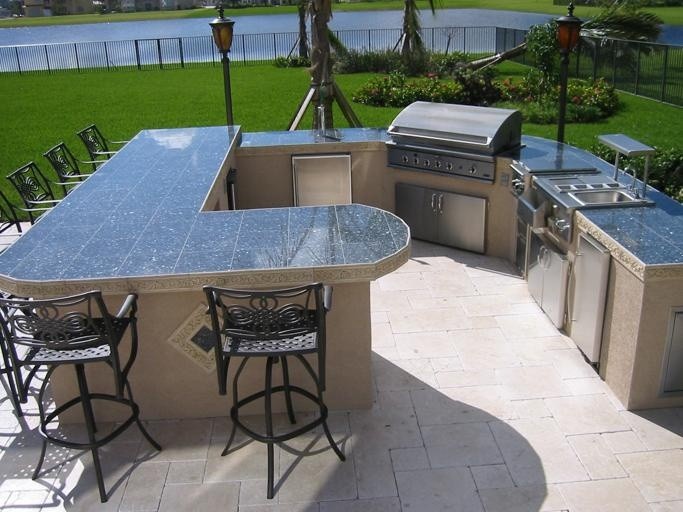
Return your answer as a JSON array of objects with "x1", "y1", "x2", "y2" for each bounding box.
[{"x1": 568, "y1": 188, "x2": 635, "y2": 206}]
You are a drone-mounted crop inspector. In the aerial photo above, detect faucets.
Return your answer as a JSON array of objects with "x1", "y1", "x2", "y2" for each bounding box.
[{"x1": 622, "y1": 165, "x2": 638, "y2": 190}]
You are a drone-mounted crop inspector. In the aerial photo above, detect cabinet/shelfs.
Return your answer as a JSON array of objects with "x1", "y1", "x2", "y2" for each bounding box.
[
  {"x1": 516, "y1": 197, "x2": 550, "y2": 276},
  {"x1": 527, "y1": 207, "x2": 570, "y2": 332},
  {"x1": 290, "y1": 152, "x2": 352, "y2": 207},
  {"x1": 565, "y1": 235, "x2": 611, "y2": 368},
  {"x1": 394, "y1": 183, "x2": 488, "y2": 257}
]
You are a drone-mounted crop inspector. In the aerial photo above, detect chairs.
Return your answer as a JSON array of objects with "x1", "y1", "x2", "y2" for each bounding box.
[
  {"x1": 200, "y1": 281, "x2": 348, "y2": 500},
  {"x1": 0, "y1": 289, "x2": 162, "y2": 505},
  {"x1": 0, "y1": 123, "x2": 130, "y2": 255}
]
